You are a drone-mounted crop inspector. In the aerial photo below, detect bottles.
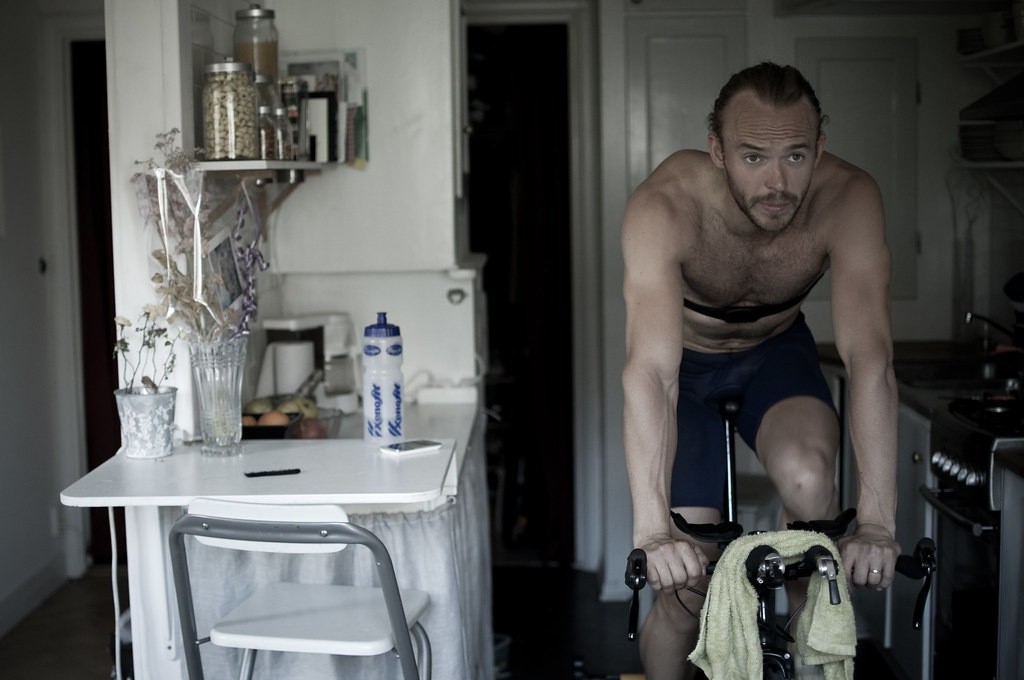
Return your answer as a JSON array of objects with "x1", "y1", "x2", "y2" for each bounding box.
[
  {"x1": 361, "y1": 312, "x2": 404, "y2": 443},
  {"x1": 328, "y1": 356, "x2": 359, "y2": 414},
  {"x1": 201, "y1": 9, "x2": 294, "y2": 162}
]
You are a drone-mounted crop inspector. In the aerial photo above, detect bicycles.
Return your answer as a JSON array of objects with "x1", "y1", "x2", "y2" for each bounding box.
[{"x1": 624, "y1": 538, "x2": 939, "y2": 680}]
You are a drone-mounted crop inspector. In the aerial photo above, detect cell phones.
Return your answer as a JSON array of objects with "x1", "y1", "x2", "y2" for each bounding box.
[{"x1": 379, "y1": 441, "x2": 441, "y2": 455}]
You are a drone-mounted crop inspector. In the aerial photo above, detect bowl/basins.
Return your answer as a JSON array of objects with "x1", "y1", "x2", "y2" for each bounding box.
[
  {"x1": 242, "y1": 411, "x2": 303, "y2": 439},
  {"x1": 295, "y1": 408, "x2": 345, "y2": 439}
]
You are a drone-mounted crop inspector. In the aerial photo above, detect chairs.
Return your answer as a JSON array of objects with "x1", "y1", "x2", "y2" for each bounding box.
[{"x1": 170, "y1": 499, "x2": 431, "y2": 680}]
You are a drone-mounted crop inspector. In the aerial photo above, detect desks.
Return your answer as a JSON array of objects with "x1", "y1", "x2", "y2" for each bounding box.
[
  {"x1": 61, "y1": 403, "x2": 490, "y2": 680},
  {"x1": 994, "y1": 448, "x2": 1024, "y2": 680}
]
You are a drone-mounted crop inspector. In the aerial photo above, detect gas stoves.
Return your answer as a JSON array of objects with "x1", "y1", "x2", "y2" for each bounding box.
[{"x1": 943, "y1": 374, "x2": 1024, "y2": 443}]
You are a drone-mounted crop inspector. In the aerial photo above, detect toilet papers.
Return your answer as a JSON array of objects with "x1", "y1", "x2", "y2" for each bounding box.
[{"x1": 255, "y1": 342, "x2": 314, "y2": 397}]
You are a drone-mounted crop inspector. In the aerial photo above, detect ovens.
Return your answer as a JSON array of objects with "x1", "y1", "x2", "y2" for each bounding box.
[{"x1": 917, "y1": 411, "x2": 1004, "y2": 680}]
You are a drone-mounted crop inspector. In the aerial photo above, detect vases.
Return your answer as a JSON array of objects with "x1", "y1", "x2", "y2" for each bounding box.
[
  {"x1": 116, "y1": 387, "x2": 176, "y2": 458},
  {"x1": 192, "y1": 341, "x2": 249, "y2": 454}
]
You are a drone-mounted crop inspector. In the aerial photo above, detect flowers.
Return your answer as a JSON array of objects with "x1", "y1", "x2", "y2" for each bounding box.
[
  {"x1": 132, "y1": 129, "x2": 247, "y2": 340},
  {"x1": 113, "y1": 301, "x2": 181, "y2": 391}
]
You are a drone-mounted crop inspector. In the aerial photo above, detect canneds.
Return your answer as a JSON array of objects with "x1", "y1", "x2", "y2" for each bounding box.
[{"x1": 275, "y1": 78, "x2": 309, "y2": 160}]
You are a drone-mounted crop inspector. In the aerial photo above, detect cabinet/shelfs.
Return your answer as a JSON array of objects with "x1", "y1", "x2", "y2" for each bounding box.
[{"x1": 819, "y1": 368, "x2": 937, "y2": 680}]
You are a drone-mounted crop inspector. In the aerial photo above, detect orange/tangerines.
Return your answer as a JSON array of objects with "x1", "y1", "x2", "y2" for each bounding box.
[
  {"x1": 242, "y1": 415, "x2": 257, "y2": 426},
  {"x1": 256, "y1": 411, "x2": 291, "y2": 426}
]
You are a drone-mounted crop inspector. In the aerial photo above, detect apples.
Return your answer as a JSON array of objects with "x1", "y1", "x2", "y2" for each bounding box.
[{"x1": 244, "y1": 396, "x2": 328, "y2": 440}]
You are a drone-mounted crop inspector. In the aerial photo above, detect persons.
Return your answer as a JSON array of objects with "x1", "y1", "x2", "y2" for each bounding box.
[{"x1": 618, "y1": 60, "x2": 900, "y2": 680}]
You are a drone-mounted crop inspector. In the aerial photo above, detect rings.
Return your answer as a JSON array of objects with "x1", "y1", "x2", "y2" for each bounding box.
[{"x1": 869, "y1": 569, "x2": 882, "y2": 574}]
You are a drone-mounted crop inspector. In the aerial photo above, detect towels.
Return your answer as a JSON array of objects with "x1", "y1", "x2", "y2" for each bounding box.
[{"x1": 685, "y1": 529, "x2": 857, "y2": 680}]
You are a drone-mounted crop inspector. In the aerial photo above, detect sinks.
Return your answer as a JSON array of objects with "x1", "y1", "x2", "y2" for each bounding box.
[{"x1": 894, "y1": 357, "x2": 982, "y2": 390}]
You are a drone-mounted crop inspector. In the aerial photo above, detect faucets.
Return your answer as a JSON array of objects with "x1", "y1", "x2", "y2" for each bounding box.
[{"x1": 964, "y1": 309, "x2": 1024, "y2": 343}]
